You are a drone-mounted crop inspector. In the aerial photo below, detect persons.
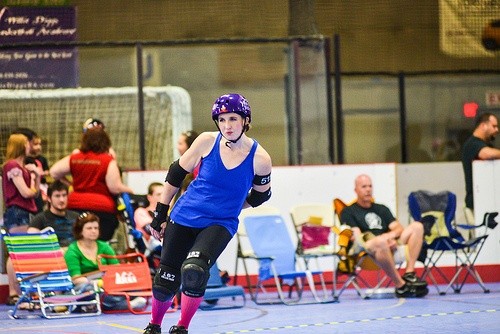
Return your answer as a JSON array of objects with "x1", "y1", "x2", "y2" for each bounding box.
[
  {"x1": 461, "y1": 113, "x2": 500, "y2": 239},
  {"x1": 1, "y1": 128, "x2": 50, "y2": 309},
  {"x1": 64, "y1": 213, "x2": 147, "y2": 313},
  {"x1": 53, "y1": 135, "x2": 132, "y2": 197},
  {"x1": 133, "y1": 183, "x2": 164, "y2": 256},
  {"x1": 26, "y1": 180, "x2": 81, "y2": 242},
  {"x1": 73, "y1": 117, "x2": 117, "y2": 158},
  {"x1": 144, "y1": 94, "x2": 272, "y2": 334},
  {"x1": 339, "y1": 174, "x2": 428, "y2": 298}
]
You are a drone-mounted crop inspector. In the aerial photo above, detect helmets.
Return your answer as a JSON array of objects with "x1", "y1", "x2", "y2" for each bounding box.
[
  {"x1": 83, "y1": 117, "x2": 104, "y2": 132},
  {"x1": 212, "y1": 94, "x2": 252, "y2": 124}
]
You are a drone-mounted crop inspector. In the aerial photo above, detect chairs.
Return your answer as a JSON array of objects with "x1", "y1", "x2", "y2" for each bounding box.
[
  {"x1": 199, "y1": 190, "x2": 499, "y2": 311},
  {"x1": 97, "y1": 252, "x2": 178, "y2": 315},
  {"x1": 0, "y1": 226, "x2": 102, "y2": 319}
]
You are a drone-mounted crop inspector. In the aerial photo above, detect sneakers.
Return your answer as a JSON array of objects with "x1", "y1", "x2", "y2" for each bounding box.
[
  {"x1": 143, "y1": 323, "x2": 161, "y2": 334},
  {"x1": 402, "y1": 272, "x2": 429, "y2": 287},
  {"x1": 169, "y1": 326, "x2": 188, "y2": 334},
  {"x1": 395, "y1": 283, "x2": 429, "y2": 298}
]
